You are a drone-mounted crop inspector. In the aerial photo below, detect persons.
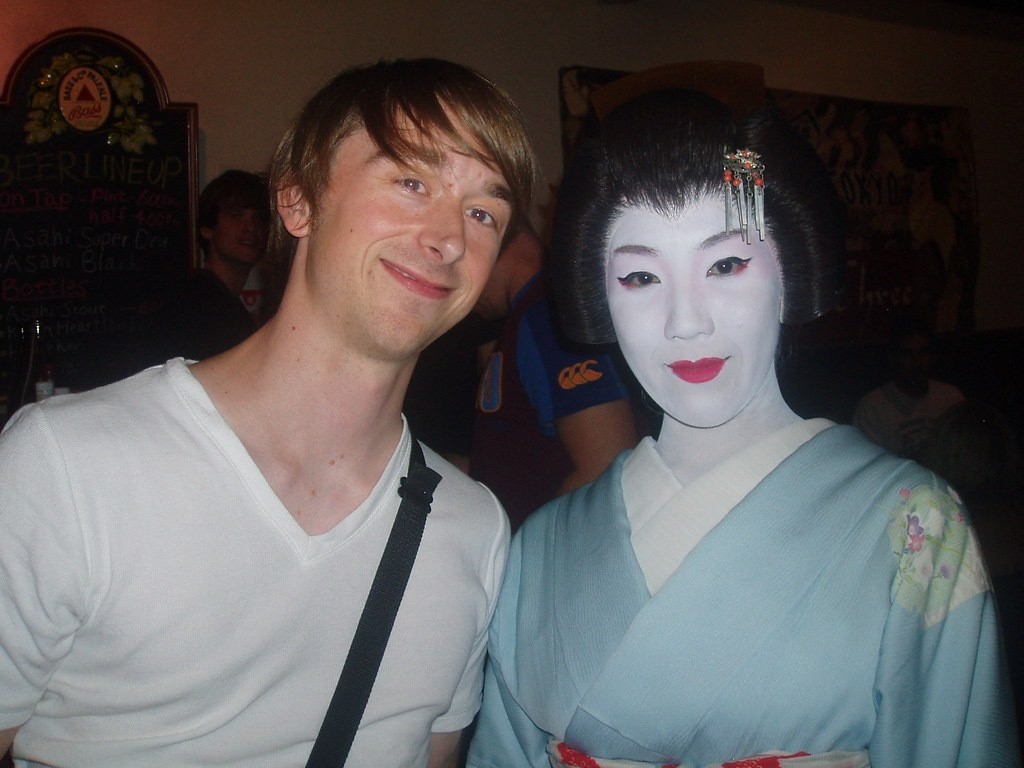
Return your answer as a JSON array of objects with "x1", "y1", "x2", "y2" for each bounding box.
[
  {"x1": 1, "y1": 58, "x2": 530, "y2": 768},
  {"x1": 462, "y1": 60, "x2": 1024, "y2": 768},
  {"x1": 139, "y1": 171, "x2": 270, "y2": 364},
  {"x1": 404, "y1": 313, "x2": 500, "y2": 475},
  {"x1": 472, "y1": 217, "x2": 654, "y2": 534},
  {"x1": 856, "y1": 317, "x2": 965, "y2": 460},
  {"x1": 924, "y1": 402, "x2": 1024, "y2": 579}
]
